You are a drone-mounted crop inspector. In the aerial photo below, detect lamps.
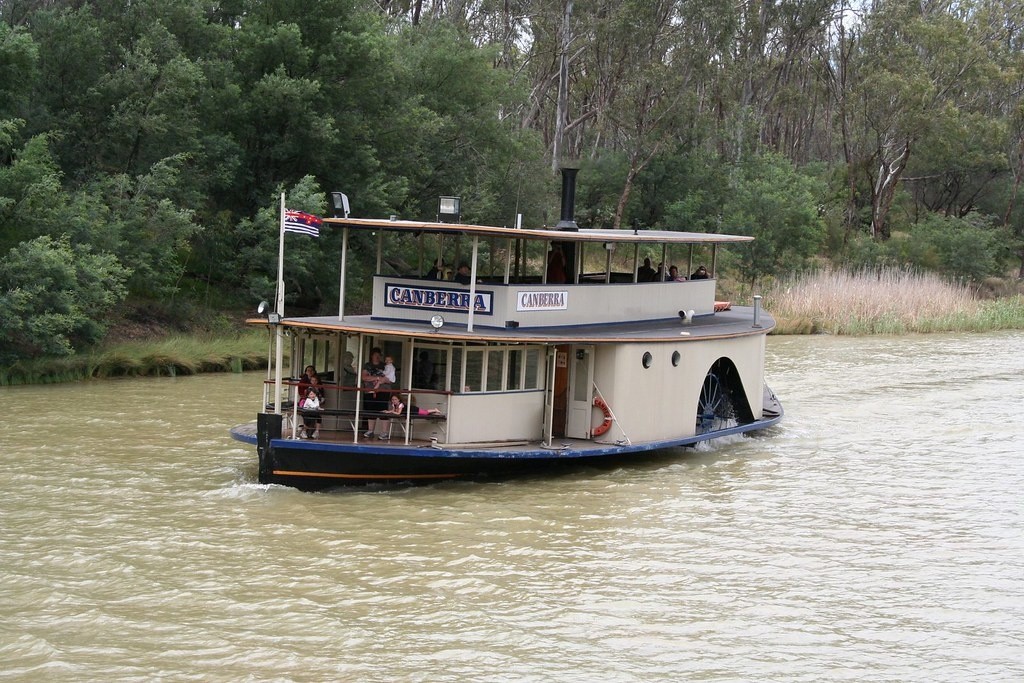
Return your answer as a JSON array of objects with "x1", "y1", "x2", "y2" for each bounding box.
[
  {"x1": 330, "y1": 192, "x2": 350, "y2": 219},
  {"x1": 431, "y1": 315, "x2": 445, "y2": 334},
  {"x1": 437, "y1": 195, "x2": 462, "y2": 224},
  {"x1": 257, "y1": 301, "x2": 269, "y2": 318}
]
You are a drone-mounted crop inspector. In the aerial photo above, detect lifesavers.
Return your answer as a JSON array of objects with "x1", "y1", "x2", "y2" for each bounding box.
[{"x1": 592, "y1": 397, "x2": 612, "y2": 439}]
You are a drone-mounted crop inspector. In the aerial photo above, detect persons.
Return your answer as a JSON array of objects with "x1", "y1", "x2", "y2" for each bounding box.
[
  {"x1": 361, "y1": 347, "x2": 396, "y2": 439},
  {"x1": 547, "y1": 250, "x2": 570, "y2": 284},
  {"x1": 638, "y1": 258, "x2": 656, "y2": 282},
  {"x1": 297, "y1": 365, "x2": 326, "y2": 439},
  {"x1": 692, "y1": 266, "x2": 708, "y2": 280},
  {"x1": 343, "y1": 351, "x2": 356, "y2": 376},
  {"x1": 380, "y1": 395, "x2": 441, "y2": 415},
  {"x1": 455, "y1": 262, "x2": 482, "y2": 285},
  {"x1": 667, "y1": 266, "x2": 687, "y2": 282},
  {"x1": 465, "y1": 384, "x2": 471, "y2": 392},
  {"x1": 653, "y1": 262, "x2": 670, "y2": 282},
  {"x1": 425, "y1": 258, "x2": 455, "y2": 281},
  {"x1": 415, "y1": 351, "x2": 436, "y2": 390}
]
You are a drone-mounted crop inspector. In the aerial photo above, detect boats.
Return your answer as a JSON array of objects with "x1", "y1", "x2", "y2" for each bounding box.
[{"x1": 228, "y1": 167, "x2": 785, "y2": 495}]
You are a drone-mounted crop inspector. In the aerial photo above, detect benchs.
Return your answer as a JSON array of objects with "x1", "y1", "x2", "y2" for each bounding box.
[{"x1": 265, "y1": 400, "x2": 447, "y2": 443}]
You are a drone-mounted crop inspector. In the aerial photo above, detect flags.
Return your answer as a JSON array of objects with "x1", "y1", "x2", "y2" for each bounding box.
[{"x1": 285, "y1": 208, "x2": 323, "y2": 237}]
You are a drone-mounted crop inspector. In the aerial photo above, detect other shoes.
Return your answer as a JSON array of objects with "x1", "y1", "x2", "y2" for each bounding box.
[
  {"x1": 312, "y1": 430, "x2": 321, "y2": 439},
  {"x1": 378, "y1": 432, "x2": 389, "y2": 440},
  {"x1": 363, "y1": 431, "x2": 375, "y2": 438},
  {"x1": 300, "y1": 430, "x2": 308, "y2": 438}
]
